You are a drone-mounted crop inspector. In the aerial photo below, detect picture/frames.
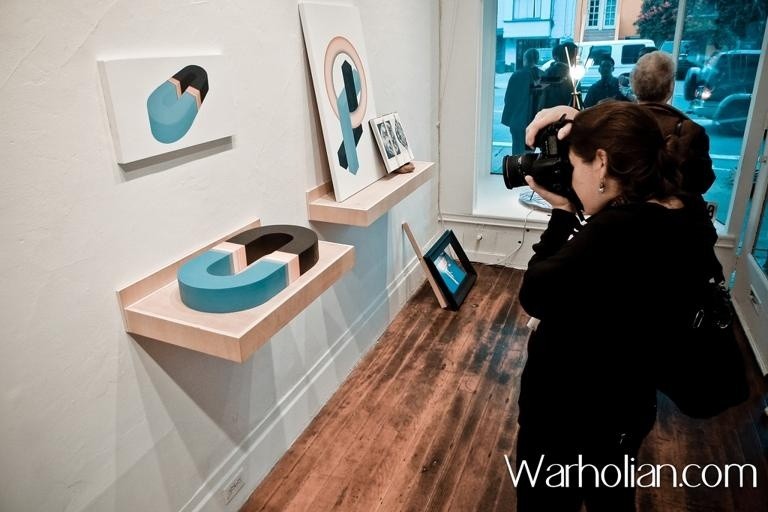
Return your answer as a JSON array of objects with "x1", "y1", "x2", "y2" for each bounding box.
[{"x1": 427, "y1": 229, "x2": 479, "y2": 311}]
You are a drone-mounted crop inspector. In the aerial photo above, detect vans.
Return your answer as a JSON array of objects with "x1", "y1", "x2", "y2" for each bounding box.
[{"x1": 574, "y1": 38, "x2": 666, "y2": 100}]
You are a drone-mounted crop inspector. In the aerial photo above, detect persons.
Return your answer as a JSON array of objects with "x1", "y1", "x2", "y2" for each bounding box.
[
  {"x1": 583, "y1": 57, "x2": 621, "y2": 108},
  {"x1": 537, "y1": 42, "x2": 578, "y2": 110},
  {"x1": 629, "y1": 51, "x2": 675, "y2": 106},
  {"x1": 501, "y1": 48, "x2": 546, "y2": 156},
  {"x1": 516, "y1": 103, "x2": 709, "y2": 512}
]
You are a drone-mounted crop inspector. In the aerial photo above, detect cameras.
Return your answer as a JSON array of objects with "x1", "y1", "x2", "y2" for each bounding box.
[{"x1": 502, "y1": 118, "x2": 572, "y2": 200}]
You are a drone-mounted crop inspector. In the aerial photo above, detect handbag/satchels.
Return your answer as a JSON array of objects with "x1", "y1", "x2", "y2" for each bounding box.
[
  {"x1": 645, "y1": 187, "x2": 751, "y2": 421},
  {"x1": 672, "y1": 118, "x2": 718, "y2": 247}
]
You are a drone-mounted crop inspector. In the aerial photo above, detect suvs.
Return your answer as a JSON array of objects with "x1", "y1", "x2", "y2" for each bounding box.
[
  {"x1": 659, "y1": 41, "x2": 694, "y2": 76},
  {"x1": 681, "y1": 50, "x2": 761, "y2": 138}
]
